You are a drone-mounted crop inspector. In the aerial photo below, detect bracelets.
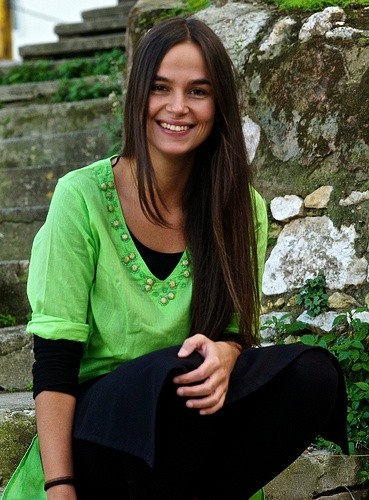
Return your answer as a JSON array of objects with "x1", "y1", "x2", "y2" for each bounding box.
[{"x1": 44, "y1": 476, "x2": 76, "y2": 491}]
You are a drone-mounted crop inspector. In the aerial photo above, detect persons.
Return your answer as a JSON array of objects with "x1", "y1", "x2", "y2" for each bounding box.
[{"x1": 0, "y1": 18, "x2": 350, "y2": 499}]
